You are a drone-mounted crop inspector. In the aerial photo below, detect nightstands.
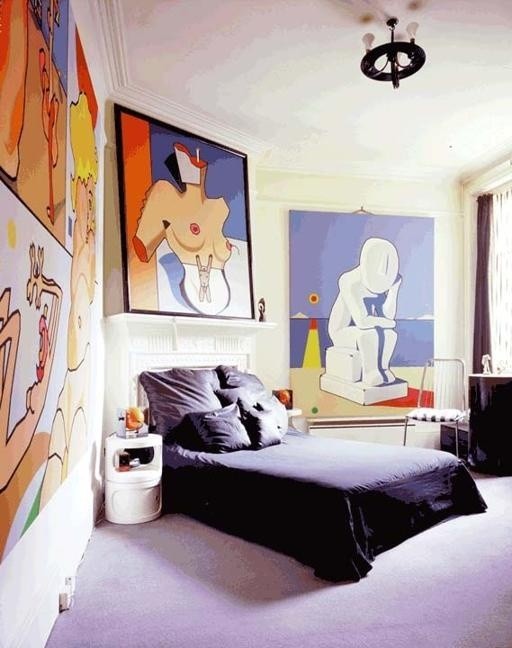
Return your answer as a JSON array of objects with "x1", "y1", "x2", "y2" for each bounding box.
[
  {"x1": 287, "y1": 408, "x2": 303, "y2": 433},
  {"x1": 105, "y1": 432, "x2": 165, "y2": 524}
]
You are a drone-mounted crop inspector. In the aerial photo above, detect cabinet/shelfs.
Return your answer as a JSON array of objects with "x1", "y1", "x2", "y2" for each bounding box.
[
  {"x1": 468, "y1": 370, "x2": 512, "y2": 475},
  {"x1": 439, "y1": 424, "x2": 468, "y2": 459}
]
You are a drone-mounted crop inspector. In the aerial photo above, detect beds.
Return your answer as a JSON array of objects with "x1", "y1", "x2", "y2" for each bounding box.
[{"x1": 128, "y1": 350, "x2": 489, "y2": 583}]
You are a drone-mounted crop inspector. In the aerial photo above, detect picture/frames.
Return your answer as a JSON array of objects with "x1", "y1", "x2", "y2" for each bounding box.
[{"x1": 112, "y1": 102, "x2": 259, "y2": 320}]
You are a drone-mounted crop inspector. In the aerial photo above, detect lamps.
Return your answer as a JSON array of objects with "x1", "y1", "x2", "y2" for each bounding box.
[{"x1": 360, "y1": 17, "x2": 427, "y2": 90}]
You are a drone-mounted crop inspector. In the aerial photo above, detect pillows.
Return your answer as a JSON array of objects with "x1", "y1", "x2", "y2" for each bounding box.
[{"x1": 138, "y1": 363, "x2": 290, "y2": 449}]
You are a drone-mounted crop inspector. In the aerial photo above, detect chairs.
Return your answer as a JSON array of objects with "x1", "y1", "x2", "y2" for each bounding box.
[{"x1": 401, "y1": 358, "x2": 469, "y2": 459}]
